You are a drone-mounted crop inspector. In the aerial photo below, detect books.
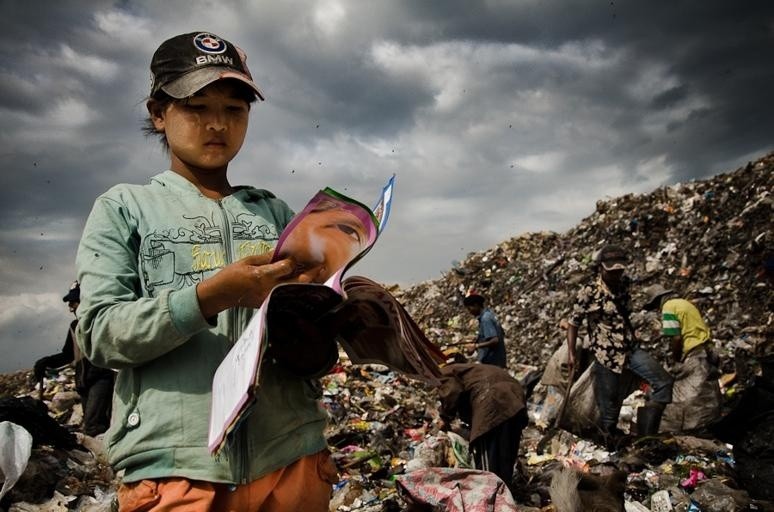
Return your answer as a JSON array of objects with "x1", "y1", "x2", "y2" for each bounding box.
[{"x1": 207, "y1": 173, "x2": 447, "y2": 458}]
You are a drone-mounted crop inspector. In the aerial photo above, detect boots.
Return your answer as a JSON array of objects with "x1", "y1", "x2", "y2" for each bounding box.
[{"x1": 637, "y1": 407, "x2": 664, "y2": 448}]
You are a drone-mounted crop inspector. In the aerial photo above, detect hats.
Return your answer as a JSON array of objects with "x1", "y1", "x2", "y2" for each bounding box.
[
  {"x1": 148, "y1": 32, "x2": 265, "y2": 101},
  {"x1": 463, "y1": 295, "x2": 485, "y2": 306},
  {"x1": 600, "y1": 246, "x2": 628, "y2": 271},
  {"x1": 63, "y1": 280, "x2": 80, "y2": 302},
  {"x1": 642, "y1": 284, "x2": 674, "y2": 311},
  {"x1": 443, "y1": 353, "x2": 466, "y2": 367}
]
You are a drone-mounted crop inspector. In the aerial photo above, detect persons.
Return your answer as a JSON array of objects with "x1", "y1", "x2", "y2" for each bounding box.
[
  {"x1": 638, "y1": 284, "x2": 714, "y2": 377},
  {"x1": 75, "y1": 32, "x2": 340, "y2": 512},
  {"x1": 436, "y1": 353, "x2": 528, "y2": 488},
  {"x1": 464, "y1": 294, "x2": 506, "y2": 368},
  {"x1": 567, "y1": 245, "x2": 674, "y2": 450},
  {"x1": 34, "y1": 282, "x2": 112, "y2": 436}
]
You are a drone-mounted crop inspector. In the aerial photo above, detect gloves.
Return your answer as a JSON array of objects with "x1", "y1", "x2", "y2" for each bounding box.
[{"x1": 35, "y1": 356, "x2": 51, "y2": 381}]
